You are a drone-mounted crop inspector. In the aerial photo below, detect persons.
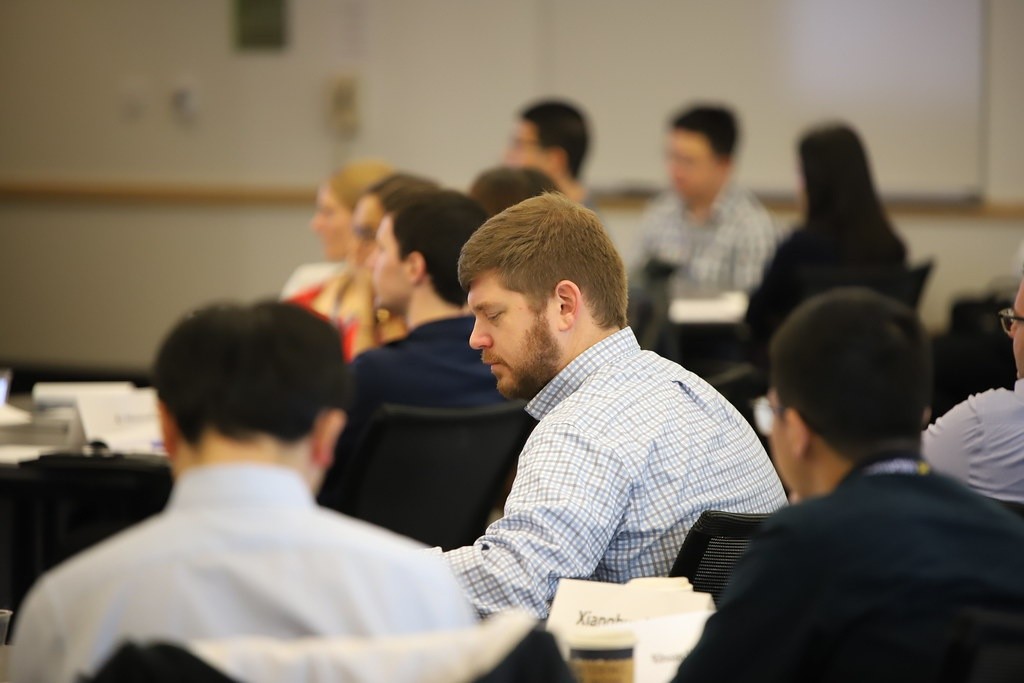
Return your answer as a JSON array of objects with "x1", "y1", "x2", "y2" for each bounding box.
[
  {"x1": 665, "y1": 287, "x2": 1024, "y2": 683},
  {"x1": 625, "y1": 101, "x2": 782, "y2": 291},
  {"x1": 284, "y1": 96, "x2": 592, "y2": 506},
  {"x1": 6, "y1": 300, "x2": 479, "y2": 683},
  {"x1": 746, "y1": 125, "x2": 908, "y2": 397},
  {"x1": 921, "y1": 278, "x2": 1024, "y2": 503},
  {"x1": 419, "y1": 192, "x2": 788, "y2": 620}
]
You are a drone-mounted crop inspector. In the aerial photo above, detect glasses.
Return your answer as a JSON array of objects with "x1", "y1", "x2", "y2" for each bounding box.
[
  {"x1": 755, "y1": 395, "x2": 794, "y2": 434},
  {"x1": 999, "y1": 308, "x2": 1024, "y2": 337}
]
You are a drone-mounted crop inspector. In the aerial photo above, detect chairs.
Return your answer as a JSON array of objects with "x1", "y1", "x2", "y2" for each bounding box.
[
  {"x1": 635, "y1": 257, "x2": 672, "y2": 350},
  {"x1": 736, "y1": 259, "x2": 932, "y2": 454},
  {"x1": 0, "y1": 463, "x2": 35, "y2": 612},
  {"x1": 331, "y1": 397, "x2": 535, "y2": 552},
  {"x1": 93, "y1": 607, "x2": 578, "y2": 683},
  {"x1": 790, "y1": 576, "x2": 1024, "y2": 683},
  {"x1": 5, "y1": 454, "x2": 172, "y2": 645},
  {"x1": 930, "y1": 295, "x2": 1016, "y2": 412},
  {"x1": 669, "y1": 510, "x2": 774, "y2": 610}
]
